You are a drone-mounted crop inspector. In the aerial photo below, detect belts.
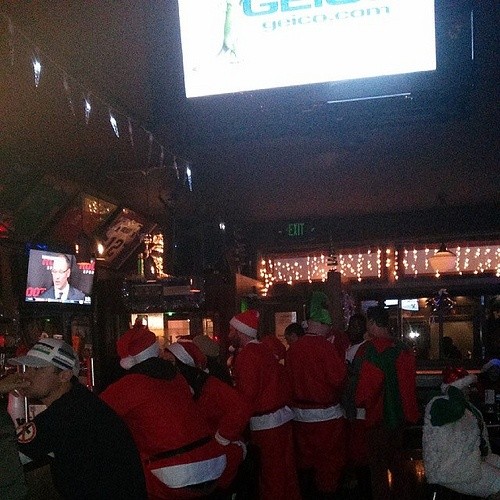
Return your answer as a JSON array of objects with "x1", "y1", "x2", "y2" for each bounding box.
[{"x1": 149, "y1": 435, "x2": 210, "y2": 461}]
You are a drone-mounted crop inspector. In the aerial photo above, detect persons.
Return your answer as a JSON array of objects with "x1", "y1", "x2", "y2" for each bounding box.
[
  {"x1": 0, "y1": 339, "x2": 151, "y2": 500},
  {"x1": 97, "y1": 302, "x2": 500, "y2": 500},
  {"x1": 40, "y1": 255, "x2": 85, "y2": 300}
]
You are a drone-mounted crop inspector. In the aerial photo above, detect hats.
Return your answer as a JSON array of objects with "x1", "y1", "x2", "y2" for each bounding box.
[
  {"x1": 116, "y1": 329, "x2": 160, "y2": 369},
  {"x1": 309, "y1": 309, "x2": 332, "y2": 324},
  {"x1": 195, "y1": 335, "x2": 220, "y2": 356},
  {"x1": 7, "y1": 338, "x2": 76, "y2": 371},
  {"x1": 177, "y1": 336, "x2": 193, "y2": 342},
  {"x1": 166, "y1": 342, "x2": 208, "y2": 369},
  {"x1": 441, "y1": 366, "x2": 477, "y2": 393},
  {"x1": 480, "y1": 348, "x2": 499, "y2": 373},
  {"x1": 229, "y1": 309, "x2": 259, "y2": 338}
]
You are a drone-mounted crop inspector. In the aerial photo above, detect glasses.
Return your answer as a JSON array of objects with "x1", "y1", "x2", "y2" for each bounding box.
[{"x1": 51, "y1": 268, "x2": 70, "y2": 274}]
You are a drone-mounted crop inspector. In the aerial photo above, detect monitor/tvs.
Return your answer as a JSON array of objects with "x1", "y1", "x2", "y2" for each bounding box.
[{"x1": 25, "y1": 249, "x2": 95, "y2": 305}]
